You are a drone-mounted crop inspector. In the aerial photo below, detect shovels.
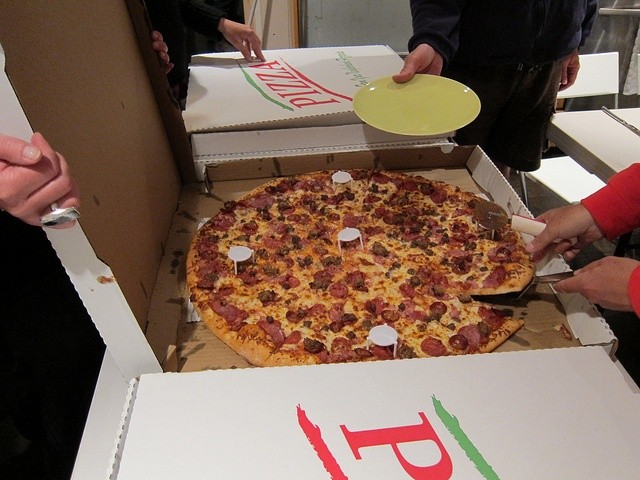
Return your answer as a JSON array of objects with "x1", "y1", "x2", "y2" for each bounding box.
[{"x1": 473, "y1": 269, "x2": 576, "y2": 302}]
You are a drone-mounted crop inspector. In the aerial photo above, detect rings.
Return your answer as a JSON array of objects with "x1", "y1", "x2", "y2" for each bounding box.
[{"x1": 39, "y1": 201, "x2": 79, "y2": 228}]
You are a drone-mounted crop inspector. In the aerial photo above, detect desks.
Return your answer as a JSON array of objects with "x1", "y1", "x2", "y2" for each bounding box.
[{"x1": 546, "y1": 108, "x2": 640, "y2": 184}]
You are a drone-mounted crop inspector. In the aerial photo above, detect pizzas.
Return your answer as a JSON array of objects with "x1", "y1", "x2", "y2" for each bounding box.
[{"x1": 187, "y1": 168, "x2": 535, "y2": 368}]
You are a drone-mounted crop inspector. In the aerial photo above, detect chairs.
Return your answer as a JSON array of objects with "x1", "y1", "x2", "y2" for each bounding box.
[{"x1": 516, "y1": 50, "x2": 625, "y2": 209}]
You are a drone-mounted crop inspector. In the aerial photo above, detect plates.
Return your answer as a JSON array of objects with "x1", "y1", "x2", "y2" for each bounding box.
[{"x1": 353, "y1": 73, "x2": 482, "y2": 135}]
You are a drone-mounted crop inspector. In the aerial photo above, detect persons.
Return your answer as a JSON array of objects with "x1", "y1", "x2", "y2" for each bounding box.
[
  {"x1": 0, "y1": 131, "x2": 79, "y2": 229},
  {"x1": 526, "y1": 161, "x2": 640, "y2": 314},
  {"x1": 146, "y1": 0, "x2": 265, "y2": 61},
  {"x1": 393, "y1": 1, "x2": 596, "y2": 170}
]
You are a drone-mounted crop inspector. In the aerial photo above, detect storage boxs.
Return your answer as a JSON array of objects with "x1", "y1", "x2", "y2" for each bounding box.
[{"x1": 191, "y1": 124, "x2": 408, "y2": 156}]
